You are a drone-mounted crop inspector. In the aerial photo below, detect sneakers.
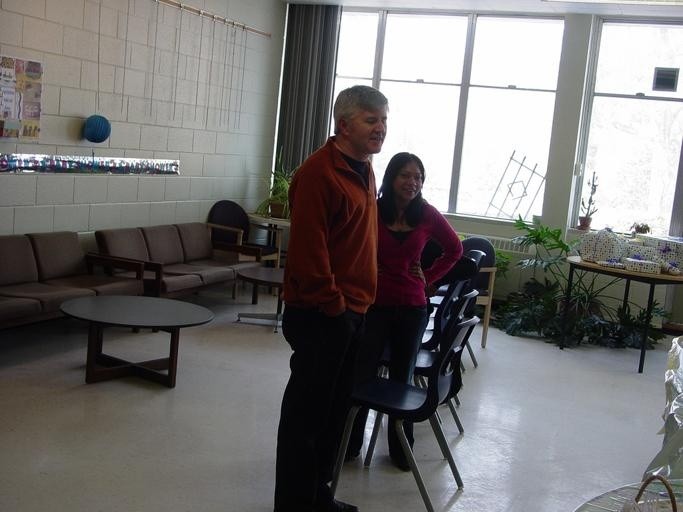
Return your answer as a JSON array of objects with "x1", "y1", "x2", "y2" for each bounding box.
[{"x1": 390, "y1": 455, "x2": 410, "y2": 471}]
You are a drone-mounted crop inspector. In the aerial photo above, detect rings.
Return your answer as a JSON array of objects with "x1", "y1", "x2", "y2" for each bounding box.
[{"x1": 417, "y1": 265, "x2": 423, "y2": 273}]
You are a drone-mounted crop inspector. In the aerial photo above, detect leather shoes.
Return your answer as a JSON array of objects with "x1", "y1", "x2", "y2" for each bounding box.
[{"x1": 329, "y1": 500, "x2": 358, "y2": 511}]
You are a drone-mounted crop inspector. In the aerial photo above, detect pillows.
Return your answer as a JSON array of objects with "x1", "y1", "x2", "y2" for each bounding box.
[{"x1": 458, "y1": 235, "x2": 495, "y2": 291}]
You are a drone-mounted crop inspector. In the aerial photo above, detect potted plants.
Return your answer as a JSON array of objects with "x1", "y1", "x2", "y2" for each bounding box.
[
  {"x1": 492, "y1": 171, "x2": 672, "y2": 350},
  {"x1": 251, "y1": 144, "x2": 300, "y2": 218}
]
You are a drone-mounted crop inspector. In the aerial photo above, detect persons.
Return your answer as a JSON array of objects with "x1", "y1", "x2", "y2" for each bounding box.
[
  {"x1": 345, "y1": 152, "x2": 463, "y2": 475},
  {"x1": 274, "y1": 86, "x2": 388, "y2": 511}
]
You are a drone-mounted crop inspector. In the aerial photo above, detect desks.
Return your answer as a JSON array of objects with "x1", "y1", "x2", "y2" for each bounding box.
[
  {"x1": 560, "y1": 256, "x2": 683, "y2": 373},
  {"x1": 247, "y1": 213, "x2": 290, "y2": 294}
]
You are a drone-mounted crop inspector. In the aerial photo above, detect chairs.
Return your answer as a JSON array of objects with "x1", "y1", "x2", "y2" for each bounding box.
[{"x1": 207, "y1": 200, "x2": 283, "y2": 296}]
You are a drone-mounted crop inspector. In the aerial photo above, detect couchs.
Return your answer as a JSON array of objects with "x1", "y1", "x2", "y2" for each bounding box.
[
  {"x1": 0, "y1": 231, "x2": 144, "y2": 333},
  {"x1": 88, "y1": 223, "x2": 263, "y2": 332}
]
